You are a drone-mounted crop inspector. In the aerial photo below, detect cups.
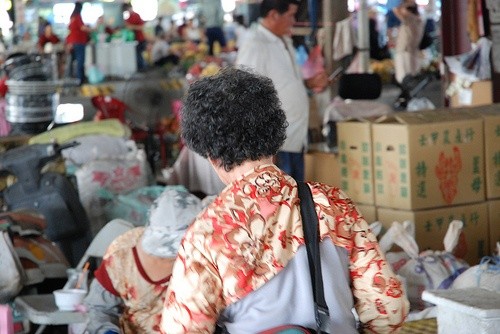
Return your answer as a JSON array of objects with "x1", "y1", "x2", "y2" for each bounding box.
[{"x1": 66, "y1": 268, "x2": 89, "y2": 292}]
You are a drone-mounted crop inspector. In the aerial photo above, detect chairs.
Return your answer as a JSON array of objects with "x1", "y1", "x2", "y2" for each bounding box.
[{"x1": 15, "y1": 218, "x2": 135, "y2": 334}]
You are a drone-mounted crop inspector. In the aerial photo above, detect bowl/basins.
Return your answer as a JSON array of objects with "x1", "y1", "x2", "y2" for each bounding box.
[{"x1": 53, "y1": 289, "x2": 87, "y2": 311}]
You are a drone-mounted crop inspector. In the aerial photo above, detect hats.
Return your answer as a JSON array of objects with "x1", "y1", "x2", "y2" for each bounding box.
[{"x1": 141, "y1": 191, "x2": 205, "y2": 258}]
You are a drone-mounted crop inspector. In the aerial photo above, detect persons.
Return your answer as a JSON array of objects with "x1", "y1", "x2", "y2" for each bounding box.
[
  {"x1": 158, "y1": 66, "x2": 412, "y2": 334},
  {"x1": 0, "y1": 0, "x2": 228, "y2": 147},
  {"x1": 393, "y1": 0, "x2": 429, "y2": 85},
  {"x1": 67, "y1": 184, "x2": 203, "y2": 334},
  {"x1": 234, "y1": 0, "x2": 327, "y2": 181}
]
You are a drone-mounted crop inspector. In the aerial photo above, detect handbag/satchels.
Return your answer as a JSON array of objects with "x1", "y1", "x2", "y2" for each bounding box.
[{"x1": 251, "y1": 324, "x2": 334, "y2": 334}]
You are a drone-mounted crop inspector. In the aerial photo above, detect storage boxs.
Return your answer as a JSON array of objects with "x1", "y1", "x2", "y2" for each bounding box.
[
  {"x1": 337, "y1": 104, "x2": 500, "y2": 266},
  {"x1": 449, "y1": 80, "x2": 493, "y2": 104},
  {"x1": 304, "y1": 151, "x2": 337, "y2": 185},
  {"x1": 421, "y1": 287, "x2": 500, "y2": 334}
]
